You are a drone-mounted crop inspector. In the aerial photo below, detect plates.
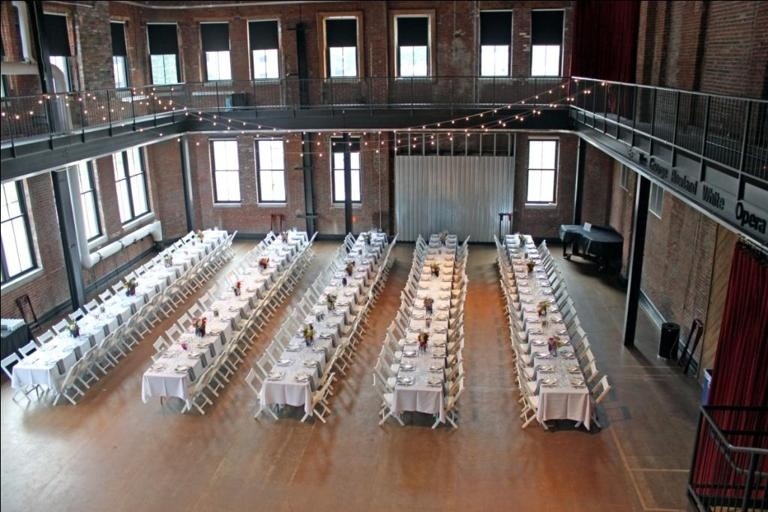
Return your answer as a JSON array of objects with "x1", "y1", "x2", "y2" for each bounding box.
[
  {"x1": 399, "y1": 233, "x2": 456, "y2": 388},
  {"x1": 152, "y1": 229, "x2": 306, "y2": 373},
  {"x1": 506, "y1": 233, "x2": 585, "y2": 388},
  {"x1": 268, "y1": 230, "x2": 385, "y2": 384},
  {"x1": 22, "y1": 226, "x2": 224, "y2": 365}
]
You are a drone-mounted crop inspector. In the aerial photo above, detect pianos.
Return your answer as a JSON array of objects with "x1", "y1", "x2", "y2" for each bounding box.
[{"x1": 559, "y1": 224, "x2": 624, "y2": 276}]
